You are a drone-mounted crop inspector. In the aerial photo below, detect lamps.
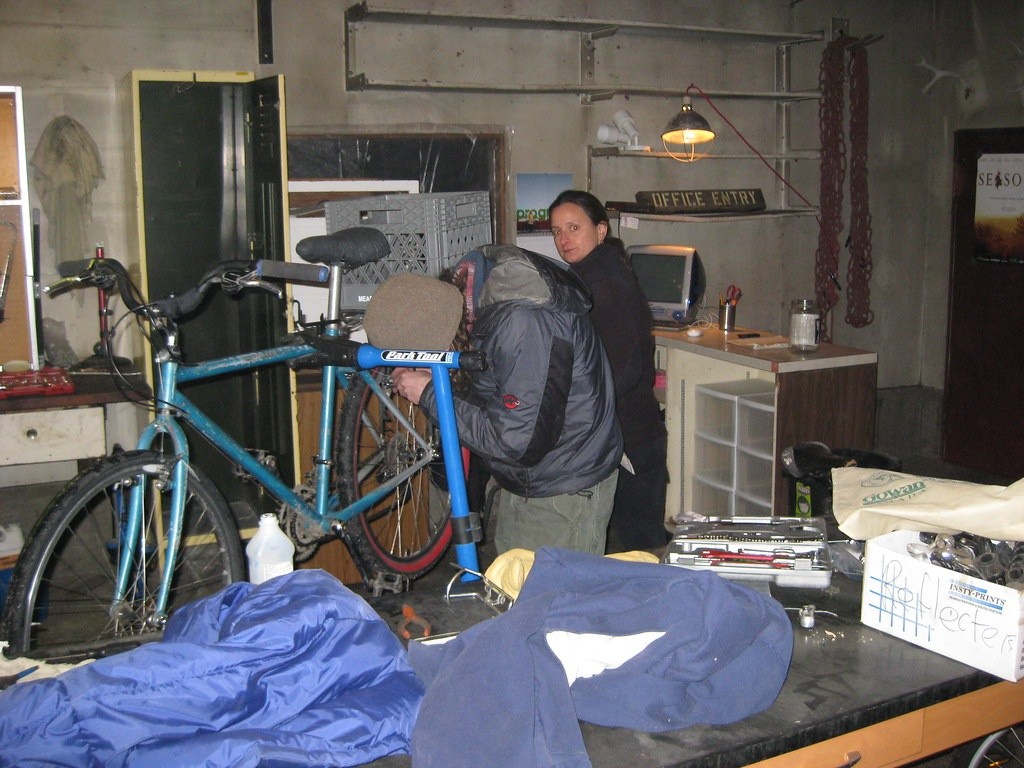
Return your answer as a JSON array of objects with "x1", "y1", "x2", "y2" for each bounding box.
[
  {"x1": 660, "y1": 96, "x2": 716, "y2": 163},
  {"x1": 596, "y1": 110, "x2": 650, "y2": 152}
]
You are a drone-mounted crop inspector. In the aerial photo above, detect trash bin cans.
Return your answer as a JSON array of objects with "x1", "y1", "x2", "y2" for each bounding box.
[{"x1": 781, "y1": 446, "x2": 902, "y2": 518}]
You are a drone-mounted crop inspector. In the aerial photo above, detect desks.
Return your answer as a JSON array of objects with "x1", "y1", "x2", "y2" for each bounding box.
[{"x1": 348, "y1": 548, "x2": 1024, "y2": 768}]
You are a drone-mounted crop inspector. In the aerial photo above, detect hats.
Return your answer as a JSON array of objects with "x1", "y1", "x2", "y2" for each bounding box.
[{"x1": 363, "y1": 272, "x2": 463, "y2": 351}]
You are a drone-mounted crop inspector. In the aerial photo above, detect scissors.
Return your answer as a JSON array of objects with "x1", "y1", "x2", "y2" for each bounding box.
[{"x1": 726, "y1": 285, "x2": 741, "y2": 300}]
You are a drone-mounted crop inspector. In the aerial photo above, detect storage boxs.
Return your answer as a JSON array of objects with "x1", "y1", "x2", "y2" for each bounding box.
[
  {"x1": 861, "y1": 529, "x2": 1024, "y2": 683},
  {"x1": 324, "y1": 191, "x2": 492, "y2": 308}
]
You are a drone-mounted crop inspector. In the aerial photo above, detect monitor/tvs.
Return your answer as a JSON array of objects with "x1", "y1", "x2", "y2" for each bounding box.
[{"x1": 625, "y1": 244, "x2": 706, "y2": 322}]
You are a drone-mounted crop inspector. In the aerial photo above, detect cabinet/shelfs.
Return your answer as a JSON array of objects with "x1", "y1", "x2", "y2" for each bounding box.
[
  {"x1": 288, "y1": 178, "x2": 432, "y2": 585},
  {"x1": 1, "y1": 84, "x2": 152, "y2": 466},
  {"x1": 344, "y1": 0, "x2": 825, "y2": 222},
  {"x1": 652, "y1": 322, "x2": 879, "y2": 531}
]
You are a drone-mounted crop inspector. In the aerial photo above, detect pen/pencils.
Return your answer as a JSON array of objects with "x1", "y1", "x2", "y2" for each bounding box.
[{"x1": 719, "y1": 290, "x2": 743, "y2": 306}]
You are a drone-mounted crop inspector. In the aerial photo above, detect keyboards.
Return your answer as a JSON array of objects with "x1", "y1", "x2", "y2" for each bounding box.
[{"x1": 648, "y1": 320, "x2": 689, "y2": 332}]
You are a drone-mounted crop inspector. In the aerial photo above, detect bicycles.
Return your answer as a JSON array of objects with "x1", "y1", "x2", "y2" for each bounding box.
[{"x1": 2, "y1": 225, "x2": 476, "y2": 664}]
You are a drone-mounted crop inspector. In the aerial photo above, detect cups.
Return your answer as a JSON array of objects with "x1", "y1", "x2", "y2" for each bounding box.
[{"x1": 718, "y1": 304, "x2": 735, "y2": 331}]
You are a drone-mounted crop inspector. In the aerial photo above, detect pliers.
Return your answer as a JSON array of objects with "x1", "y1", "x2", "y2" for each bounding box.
[{"x1": 398, "y1": 602, "x2": 431, "y2": 639}]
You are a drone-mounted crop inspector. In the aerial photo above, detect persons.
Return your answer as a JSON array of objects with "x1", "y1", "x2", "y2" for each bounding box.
[
  {"x1": 549, "y1": 188, "x2": 671, "y2": 552},
  {"x1": 363, "y1": 243, "x2": 623, "y2": 559},
  {"x1": 527, "y1": 210, "x2": 535, "y2": 230}
]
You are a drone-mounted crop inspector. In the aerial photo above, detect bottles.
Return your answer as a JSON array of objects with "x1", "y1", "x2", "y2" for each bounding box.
[
  {"x1": 789, "y1": 299, "x2": 821, "y2": 352},
  {"x1": 0, "y1": 523, "x2": 26, "y2": 626},
  {"x1": 246, "y1": 513, "x2": 295, "y2": 587}
]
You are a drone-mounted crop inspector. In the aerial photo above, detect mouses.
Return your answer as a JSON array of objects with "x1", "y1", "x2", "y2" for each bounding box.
[{"x1": 686, "y1": 325, "x2": 703, "y2": 337}]
